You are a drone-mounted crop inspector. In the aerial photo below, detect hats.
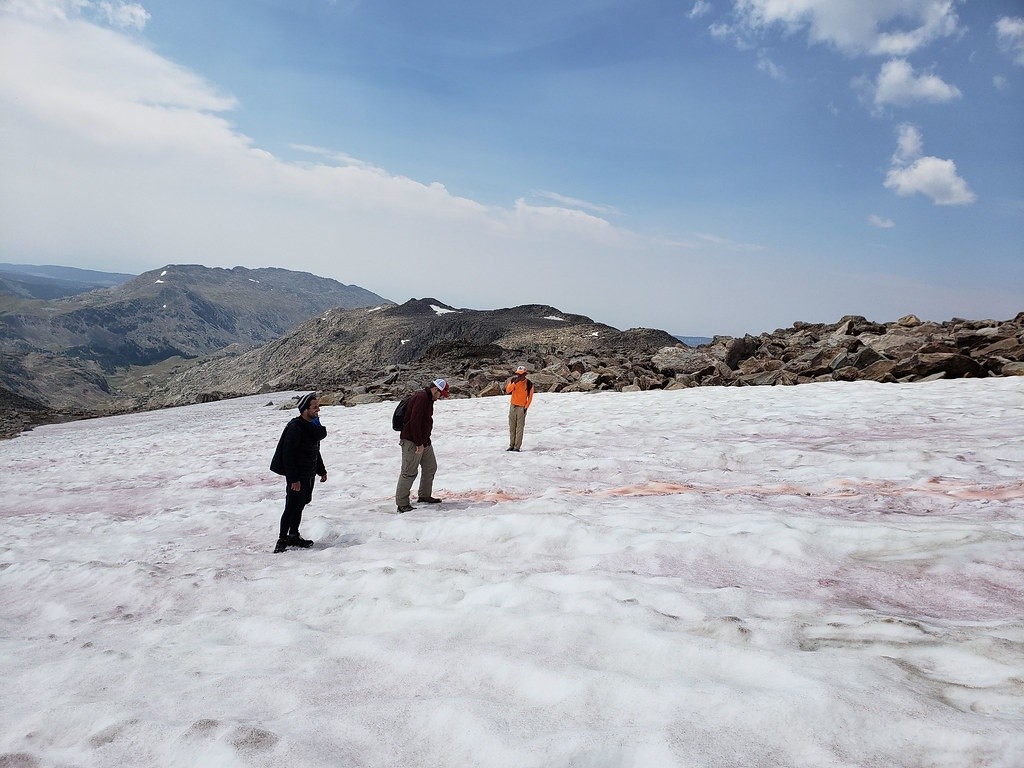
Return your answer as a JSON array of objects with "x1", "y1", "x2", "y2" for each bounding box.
[
  {"x1": 432, "y1": 378, "x2": 449, "y2": 398},
  {"x1": 297, "y1": 395, "x2": 314, "y2": 414},
  {"x1": 515, "y1": 366, "x2": 527, "y2": 375}
]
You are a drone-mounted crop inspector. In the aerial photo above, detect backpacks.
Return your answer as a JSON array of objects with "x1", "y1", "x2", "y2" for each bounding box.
[{"x1": 392, "y1": 393, "x2": 415, "y2": 430}]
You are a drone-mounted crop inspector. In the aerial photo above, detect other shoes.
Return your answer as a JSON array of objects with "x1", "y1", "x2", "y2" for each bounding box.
[
  {"x1": 274, "y1": 537, "x2": 289, "y2": 553},
  {"x1": 417, "y1": 496, "x2": 442, "y2": 504},
  {"x1": 512, "y1": 448, "x2": 519, "y2": 451},
  {"x1": 507, "y1": 447, "x2": 514, "y2": 451},
  {"x1": 397, "y1": 505, "x2": 417, "y2": 513},
  {"x1": 287, "y1": 535, "x2": 314, "y2": 548}
]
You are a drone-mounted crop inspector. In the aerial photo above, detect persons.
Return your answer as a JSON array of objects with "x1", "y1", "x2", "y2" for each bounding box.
[
  {"x1": 270, "y1": 394, "x2": 328, "y2": 553},
  {"x1": 395, "y1": 379, "x2": 449, "y2": 513},
  {"x1": 506, "y1": 366, "x2": 534, "y2": 452}
]
